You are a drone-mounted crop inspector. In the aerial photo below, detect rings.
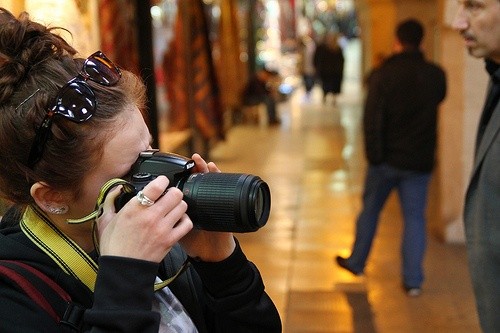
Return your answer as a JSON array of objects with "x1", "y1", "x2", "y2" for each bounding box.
[{"x1": 137, "y1": 191, "x2": 154, "y2": 206}]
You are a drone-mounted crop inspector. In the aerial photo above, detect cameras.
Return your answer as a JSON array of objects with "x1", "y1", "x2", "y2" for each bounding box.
[{"x1": 115, "y1": 149, "x2": 272, "y2": 232}]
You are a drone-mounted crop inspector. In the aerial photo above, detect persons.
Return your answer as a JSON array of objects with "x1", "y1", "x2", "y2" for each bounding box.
[
  {"x1": 238, "y1": 21, "x2": 345, "y2": 130},
  {"x1": 0, "y1": 8, "x2": 282, "y2": 333},
  {"x1": 450, "y1": 0, "x2": 500, "y2": 333},
  {"x1": 336, "y1": 17, "x2": 447, "y2": 295}
]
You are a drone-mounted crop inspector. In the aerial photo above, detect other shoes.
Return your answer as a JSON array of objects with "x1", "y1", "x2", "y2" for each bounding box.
[
  {"x1": 402, "y1": 283, "x2": 423, "y2": 297},
  {"x1": 335, "y1": 255, "x2": 360, "y2": 277}
]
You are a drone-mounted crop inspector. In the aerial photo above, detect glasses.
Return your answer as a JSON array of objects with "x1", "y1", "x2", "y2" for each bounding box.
[{"x1": 27, "y1": 50, "x2": 123, "y2": 170}]
